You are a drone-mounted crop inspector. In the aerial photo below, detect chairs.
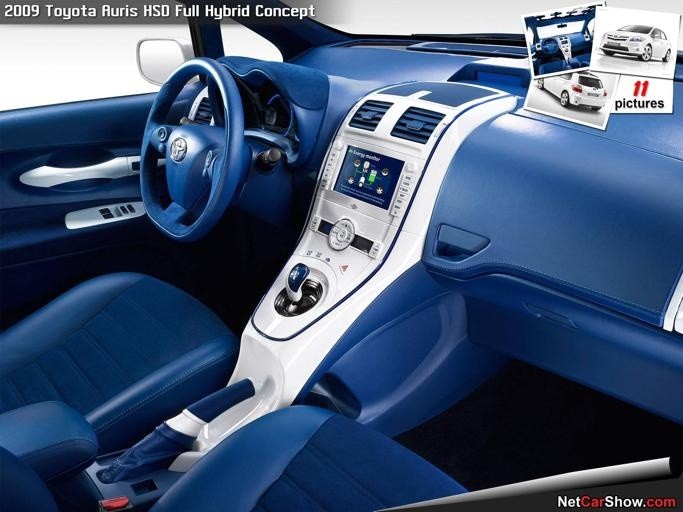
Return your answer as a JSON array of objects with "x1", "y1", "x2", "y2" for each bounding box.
[
  {"x1": 0, "y1": 271, "x2": 239, "y2": 463},
  {"x1": 0, "y1": 396, "x2": 468, "y2": 511}
]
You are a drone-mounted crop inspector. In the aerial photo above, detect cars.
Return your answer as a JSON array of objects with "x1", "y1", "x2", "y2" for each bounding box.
[{"x1": 599, "y1": 24, "x2": 671, "y2": 62}]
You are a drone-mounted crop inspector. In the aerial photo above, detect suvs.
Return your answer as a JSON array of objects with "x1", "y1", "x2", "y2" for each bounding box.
[{"x1": 538, "y1": 72, "x2": 606, "y2": 112}]
